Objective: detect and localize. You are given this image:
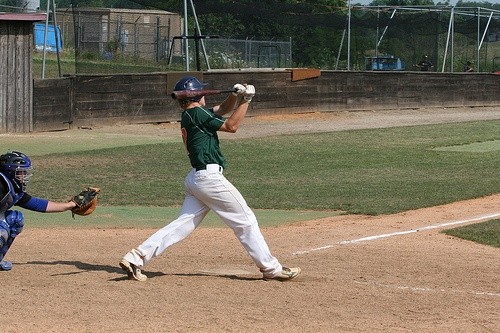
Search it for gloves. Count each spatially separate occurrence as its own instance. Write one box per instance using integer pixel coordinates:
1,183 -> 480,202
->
243,84 -> 255,101
232,83 -> 246,97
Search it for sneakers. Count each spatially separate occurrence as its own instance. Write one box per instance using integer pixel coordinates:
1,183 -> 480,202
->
119,258 -> 147,281
263,266 -> 301,281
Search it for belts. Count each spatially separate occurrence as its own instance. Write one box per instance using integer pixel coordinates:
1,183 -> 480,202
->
195,166 -> 221,172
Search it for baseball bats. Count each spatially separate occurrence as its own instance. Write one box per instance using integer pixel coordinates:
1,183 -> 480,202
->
170,87 -> 237,98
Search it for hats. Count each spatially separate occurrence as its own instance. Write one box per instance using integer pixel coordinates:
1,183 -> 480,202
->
0,153 -> 18,172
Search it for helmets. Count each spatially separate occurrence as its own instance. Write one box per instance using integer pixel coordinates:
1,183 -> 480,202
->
175,77 -> 208,105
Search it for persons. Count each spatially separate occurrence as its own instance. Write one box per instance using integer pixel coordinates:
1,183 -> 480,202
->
414,55 -> 434,71
464,61 -> 473,72
119,77 -> 301,282
0,151 -> 100,270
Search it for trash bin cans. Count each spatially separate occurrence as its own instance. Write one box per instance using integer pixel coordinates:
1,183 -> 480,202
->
33,24 -> 63,54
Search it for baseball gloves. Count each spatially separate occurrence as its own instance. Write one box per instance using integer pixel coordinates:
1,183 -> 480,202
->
69,186 -> 100,215
